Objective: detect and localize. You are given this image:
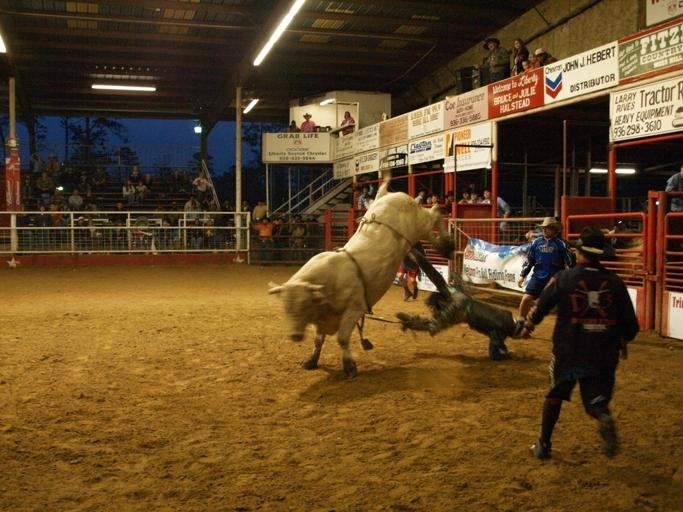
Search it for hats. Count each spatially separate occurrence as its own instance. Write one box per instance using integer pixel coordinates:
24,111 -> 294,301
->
538,217 -> 563,231
577,226 -> 606,254
482,38 -> 500,50
303,113 -> 312,118
534,47 -> 546,55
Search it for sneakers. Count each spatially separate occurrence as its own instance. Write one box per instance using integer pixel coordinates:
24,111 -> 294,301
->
531,438 -> 553,459
598,415 -> 620,454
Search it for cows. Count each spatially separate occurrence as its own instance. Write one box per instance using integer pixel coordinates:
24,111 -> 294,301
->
266,168 -> 457,379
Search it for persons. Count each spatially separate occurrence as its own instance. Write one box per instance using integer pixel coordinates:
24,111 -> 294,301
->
251,213 -> 306,261
514,217 -> 572,337
414,189 -> 511,232
354,174 -> 376,212
664,166 -> 683,213
395,249 -> 525,360
341,111 -> 355,136
473,33 -> 557,86
301,113 -> 317,133
13,153 -> 267,250
521,228 -> 640,460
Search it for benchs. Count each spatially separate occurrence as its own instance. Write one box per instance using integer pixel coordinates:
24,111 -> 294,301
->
20,175 -> 197,245
